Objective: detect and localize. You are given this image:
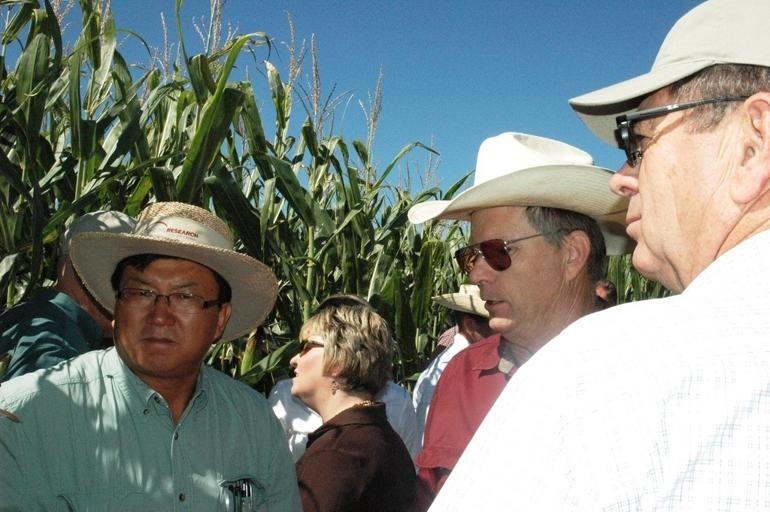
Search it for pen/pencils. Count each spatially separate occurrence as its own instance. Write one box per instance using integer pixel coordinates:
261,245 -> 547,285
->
228,479 -> 254,512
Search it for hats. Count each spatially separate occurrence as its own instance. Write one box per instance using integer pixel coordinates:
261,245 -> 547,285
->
569,0 -> 770,149
431,282 -> 490,317
408,130 -> 638,256
61,201 -> 278,344
318,293 -> 374,311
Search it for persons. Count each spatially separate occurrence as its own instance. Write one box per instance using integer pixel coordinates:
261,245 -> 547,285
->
0,195 -> 309,511
410,282 -> 499,448
264,290 -> 422,486
285,291 -> 422,511
420,1 -> 770,512
405,129 -> 640,511
1,208 -> 141,386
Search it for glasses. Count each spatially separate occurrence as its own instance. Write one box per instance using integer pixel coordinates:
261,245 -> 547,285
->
615,96 -> 749,166
455,228 -> 568,276
116,289 -> 221,313
297,341 -> 323,351
